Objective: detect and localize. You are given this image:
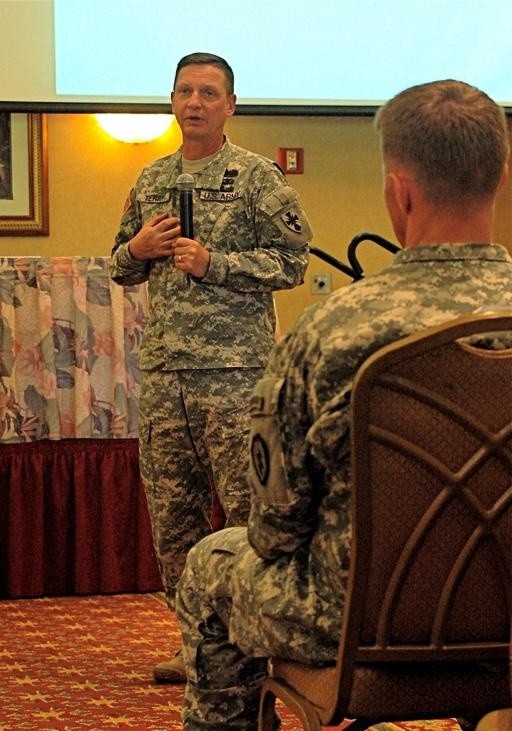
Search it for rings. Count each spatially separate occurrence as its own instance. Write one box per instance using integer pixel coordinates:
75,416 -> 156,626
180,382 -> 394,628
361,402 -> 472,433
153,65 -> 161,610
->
177,253 -> 181,263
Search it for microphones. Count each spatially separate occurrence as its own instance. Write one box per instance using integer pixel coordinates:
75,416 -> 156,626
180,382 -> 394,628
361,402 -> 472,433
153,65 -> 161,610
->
175,173 -> 197,240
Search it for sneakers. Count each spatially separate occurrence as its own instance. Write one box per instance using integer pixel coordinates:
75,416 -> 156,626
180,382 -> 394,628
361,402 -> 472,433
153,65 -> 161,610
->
154,650 -> 187,683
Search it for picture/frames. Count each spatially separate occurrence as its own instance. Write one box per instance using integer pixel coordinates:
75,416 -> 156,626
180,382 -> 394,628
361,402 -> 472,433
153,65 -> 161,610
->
1,112 -> 54,240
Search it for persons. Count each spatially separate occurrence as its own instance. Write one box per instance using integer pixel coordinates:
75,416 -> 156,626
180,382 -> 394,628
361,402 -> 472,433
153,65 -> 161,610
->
175,77 -> 512,730
107,50 -> 318,683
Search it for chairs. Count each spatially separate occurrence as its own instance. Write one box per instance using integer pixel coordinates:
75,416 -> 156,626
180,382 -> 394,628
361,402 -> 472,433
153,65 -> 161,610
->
252,312 -> 508,728
